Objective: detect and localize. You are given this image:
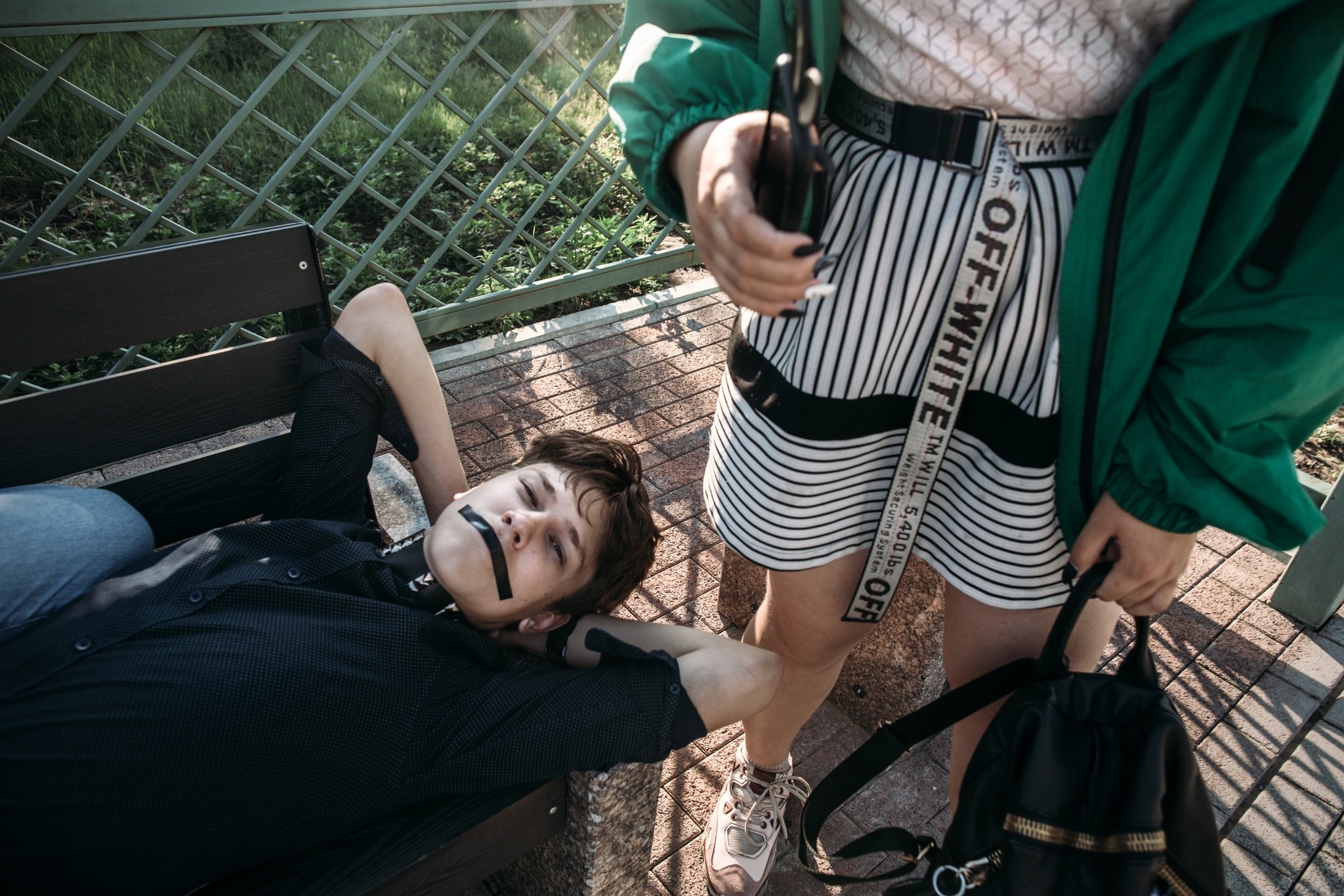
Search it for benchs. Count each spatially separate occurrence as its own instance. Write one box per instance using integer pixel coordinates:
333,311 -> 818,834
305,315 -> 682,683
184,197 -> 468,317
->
0,217 -> 382,546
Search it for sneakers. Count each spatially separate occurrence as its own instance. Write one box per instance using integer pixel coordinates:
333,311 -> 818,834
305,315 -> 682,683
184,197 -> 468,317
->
700,739 -> 812,896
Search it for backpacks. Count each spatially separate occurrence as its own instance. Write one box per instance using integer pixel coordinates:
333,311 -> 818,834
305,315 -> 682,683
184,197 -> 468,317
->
793,556 -> 1225,896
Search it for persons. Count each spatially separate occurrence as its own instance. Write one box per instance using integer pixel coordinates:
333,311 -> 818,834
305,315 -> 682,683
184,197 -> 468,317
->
0,282 -> 776,896
607,0 -> 1344,896
